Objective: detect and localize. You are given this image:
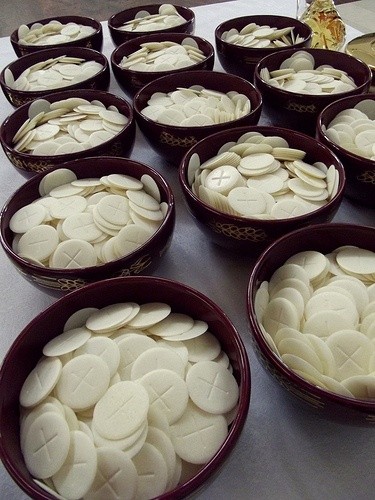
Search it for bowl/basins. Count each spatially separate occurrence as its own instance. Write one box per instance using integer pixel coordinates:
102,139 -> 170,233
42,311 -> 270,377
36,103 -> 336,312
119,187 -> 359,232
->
0,275 -> 250,500
178,124 -> 345,252
0,47 -> 111,110
132,69 -> 263,164
0,156 -> 175,303
245,222 -> 375,429
0,86 -> 139,183
314,92 -> 375,210
9,15 -> 104,58
106,4 -> 196,50
214,14 -> 312,88
254,47 -> 373,137
108,32 -> 215,100
